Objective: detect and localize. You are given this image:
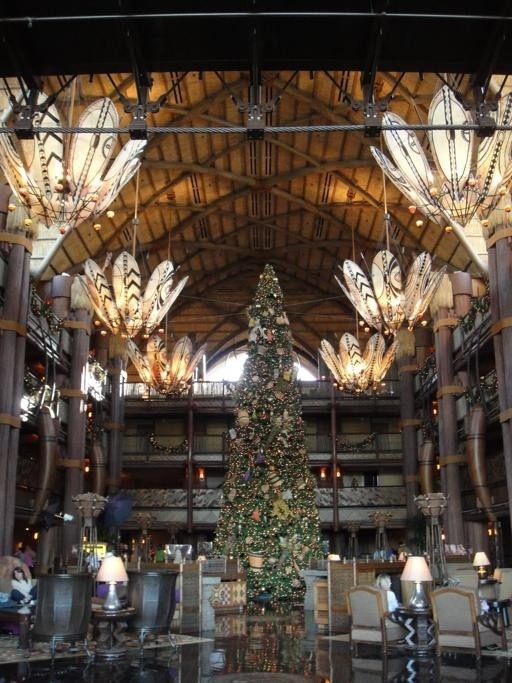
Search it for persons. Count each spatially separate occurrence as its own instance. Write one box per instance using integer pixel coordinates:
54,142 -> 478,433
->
11,567 -> 37,606
48,557 -> 67,573
19,542 -> 37,579
478,584 -> 499,615
376,573 -> 402,621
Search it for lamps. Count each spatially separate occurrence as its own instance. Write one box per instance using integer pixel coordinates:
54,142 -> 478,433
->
316,208 -> 401,395
76,167 -> 190,339
97,556 -> 130,611
0,75 -> 148,235
399,555 -> 434,612
126,207 -> 207,397
369,71 -> 512,233
472,551 -> 492,581
331,128 -> 447,335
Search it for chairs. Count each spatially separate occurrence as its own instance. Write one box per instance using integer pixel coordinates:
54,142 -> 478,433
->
299,561 -> 512,681
